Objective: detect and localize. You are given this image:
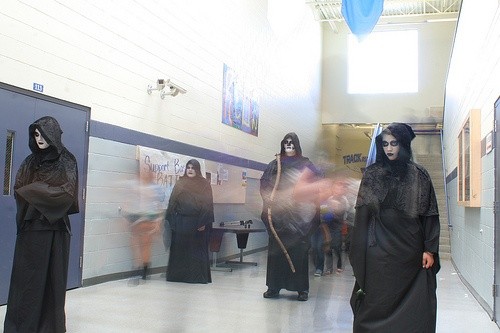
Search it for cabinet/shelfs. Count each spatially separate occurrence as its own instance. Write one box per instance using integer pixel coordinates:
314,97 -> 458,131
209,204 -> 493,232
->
456,108 -> 481,207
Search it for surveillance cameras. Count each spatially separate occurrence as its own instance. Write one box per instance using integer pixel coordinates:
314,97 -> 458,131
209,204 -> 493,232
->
157,79 -> 165,90
165,79 -> 187,94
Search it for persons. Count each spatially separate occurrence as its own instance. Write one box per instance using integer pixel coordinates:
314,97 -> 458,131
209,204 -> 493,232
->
164,159 -> 215,284
4,116 -> 81,333
349,122 -> 441,333
260,132 -> 319,301
311,183 -> 349,275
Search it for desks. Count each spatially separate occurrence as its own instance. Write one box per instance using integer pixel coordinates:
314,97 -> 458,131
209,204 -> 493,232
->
211,226 -> 266,272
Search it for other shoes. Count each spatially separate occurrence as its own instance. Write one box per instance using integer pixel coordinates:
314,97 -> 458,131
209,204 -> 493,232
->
337,269 -> 342,272
314,269 -> 323,276
325,270 -> 333,274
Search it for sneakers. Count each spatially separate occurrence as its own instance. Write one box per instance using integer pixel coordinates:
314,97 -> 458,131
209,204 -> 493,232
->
263,289 -> 279,298
298,291 -> 308,301
